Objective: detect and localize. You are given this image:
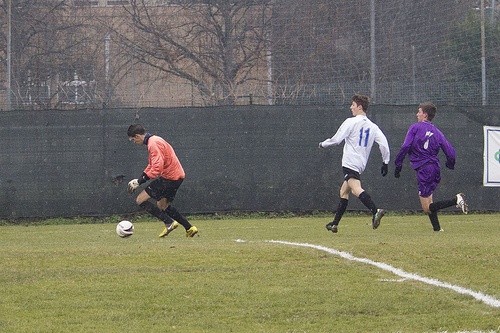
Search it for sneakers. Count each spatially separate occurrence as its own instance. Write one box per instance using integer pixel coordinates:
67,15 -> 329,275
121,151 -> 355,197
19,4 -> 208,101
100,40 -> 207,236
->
456,193 -> 469,214
372,208 -> 384,230
160,221 -> 179,238
186,226 -> 198,238
326,223 -> 337,233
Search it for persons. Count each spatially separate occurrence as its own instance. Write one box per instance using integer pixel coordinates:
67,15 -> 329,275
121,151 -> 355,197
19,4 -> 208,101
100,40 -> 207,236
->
319,95 -> 390,233
395,102 -> 468,231
127,124 -> 197,237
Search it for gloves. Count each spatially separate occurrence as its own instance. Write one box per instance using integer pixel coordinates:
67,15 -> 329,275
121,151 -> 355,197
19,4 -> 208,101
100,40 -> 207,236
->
394,167 -> 401,178
381,163 -> 388,176
127,179 -> 139,193
446,162 -> 456,169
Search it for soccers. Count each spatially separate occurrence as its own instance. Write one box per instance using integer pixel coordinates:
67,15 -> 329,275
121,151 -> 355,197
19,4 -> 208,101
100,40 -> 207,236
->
116,220 -> 134,238
